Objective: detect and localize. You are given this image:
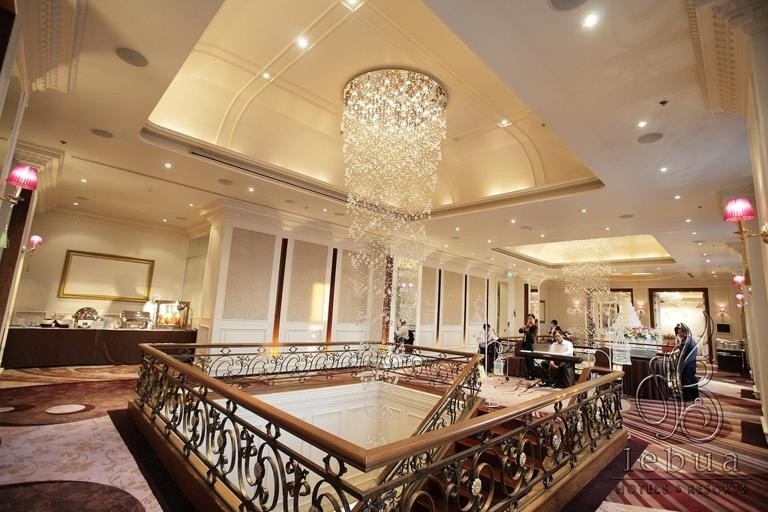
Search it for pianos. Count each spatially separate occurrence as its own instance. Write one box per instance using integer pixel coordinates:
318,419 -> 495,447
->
520,350 -> 584,363
657,349 -> 680,357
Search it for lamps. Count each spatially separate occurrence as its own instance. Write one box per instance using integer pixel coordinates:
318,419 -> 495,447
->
340,68 -> 450,331
718,197 -> 768,318
552,238 -> 613,348
0,163 -> 40,205
22,235 -> 43,253
177,304 -> 193,330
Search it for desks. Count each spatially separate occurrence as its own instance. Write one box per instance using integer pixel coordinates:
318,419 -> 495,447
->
0,327 -> 197,369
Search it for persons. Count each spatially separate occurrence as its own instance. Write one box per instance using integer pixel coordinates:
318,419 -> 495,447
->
548,320 -> 566,343
478,324 -> 499,373
674,324 -> 698,401
395,318 -> 413,345
519,313 -> 538,380
541,330 -> 574,384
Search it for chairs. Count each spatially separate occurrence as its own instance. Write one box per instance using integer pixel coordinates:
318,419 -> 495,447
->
593,347 -> 613,390
504,339 -> 528,377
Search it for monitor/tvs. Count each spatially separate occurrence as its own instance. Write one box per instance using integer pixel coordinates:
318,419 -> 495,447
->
717,323 -> 730,332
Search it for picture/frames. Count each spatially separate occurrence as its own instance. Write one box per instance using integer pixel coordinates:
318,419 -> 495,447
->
57,248 -> 155,303
